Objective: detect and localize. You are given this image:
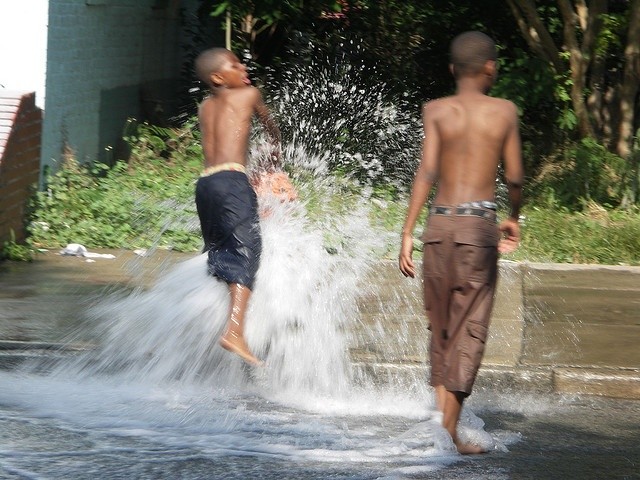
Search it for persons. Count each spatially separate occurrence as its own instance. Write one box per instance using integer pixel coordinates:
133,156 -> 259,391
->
192,47 -> 284,368
398,31 -> 524,456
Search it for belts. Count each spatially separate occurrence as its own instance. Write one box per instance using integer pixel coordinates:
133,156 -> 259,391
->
429,205 -> 497,221
